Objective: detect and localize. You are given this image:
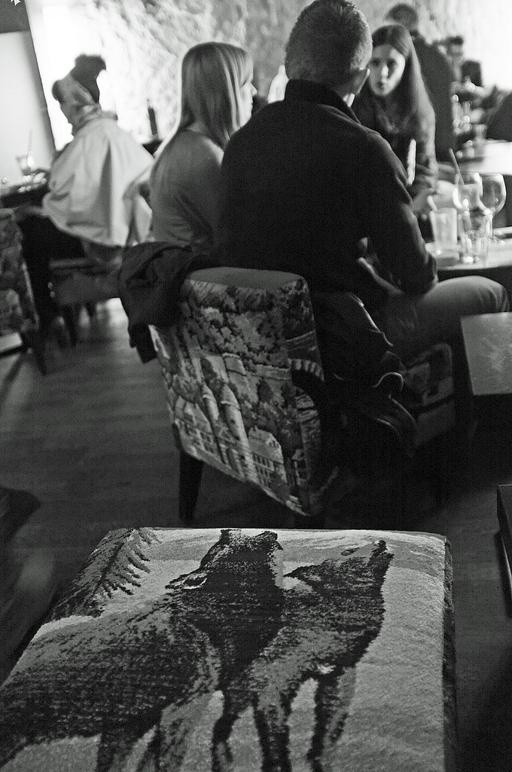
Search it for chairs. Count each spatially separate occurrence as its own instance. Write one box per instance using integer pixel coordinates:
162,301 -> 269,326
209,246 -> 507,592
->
144,247 -> 480,528
0,182 -> 165,377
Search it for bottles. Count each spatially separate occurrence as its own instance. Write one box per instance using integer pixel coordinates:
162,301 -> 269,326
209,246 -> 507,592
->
142,99 -> 165,139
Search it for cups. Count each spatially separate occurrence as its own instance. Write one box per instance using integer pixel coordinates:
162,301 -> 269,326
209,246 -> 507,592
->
15,150 -> 41,187
428,168 -> 508,265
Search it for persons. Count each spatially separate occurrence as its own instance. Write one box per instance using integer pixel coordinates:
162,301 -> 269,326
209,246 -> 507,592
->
15,52 -> 157,343
151,2 -> 512,488
